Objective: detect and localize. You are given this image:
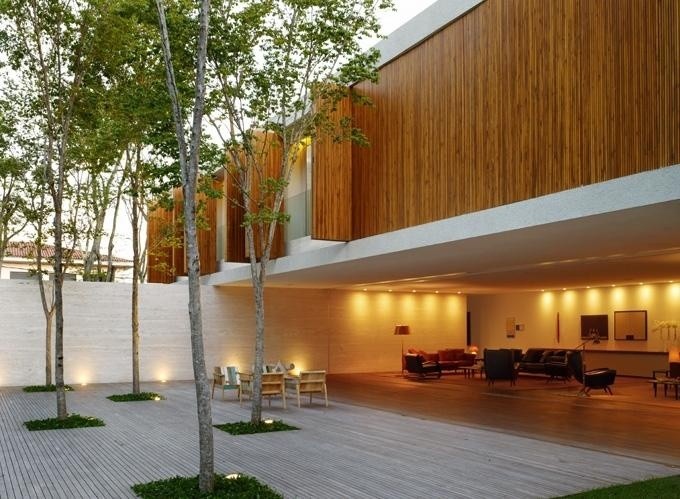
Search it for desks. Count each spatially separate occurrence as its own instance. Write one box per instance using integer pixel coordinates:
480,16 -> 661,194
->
238,371 -> 253,398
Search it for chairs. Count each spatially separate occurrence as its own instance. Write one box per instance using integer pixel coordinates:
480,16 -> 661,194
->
290,368 -> 330,410
256,373 -> 288,411
211,366 -> 241,402
253,363 -> 290,399
402,346 -> 680,403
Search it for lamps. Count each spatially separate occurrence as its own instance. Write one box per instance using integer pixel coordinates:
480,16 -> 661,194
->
393,325 -> 412,377
